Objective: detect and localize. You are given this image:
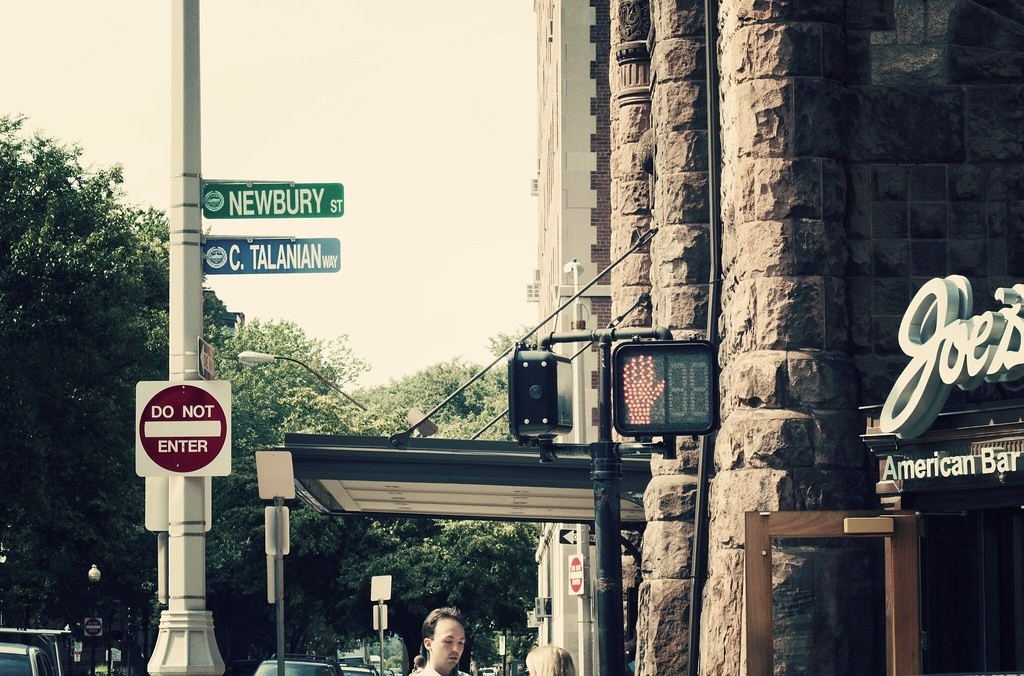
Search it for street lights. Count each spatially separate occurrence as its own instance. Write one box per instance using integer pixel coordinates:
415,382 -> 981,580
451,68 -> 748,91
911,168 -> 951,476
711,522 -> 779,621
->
88,565 -> 101,676
234,348 -> 372,415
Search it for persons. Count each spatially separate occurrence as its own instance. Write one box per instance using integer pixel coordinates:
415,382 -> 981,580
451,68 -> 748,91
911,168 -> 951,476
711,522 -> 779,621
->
408,607 -> 470,676
408,656 -> 427,676
526,646 -> 575,676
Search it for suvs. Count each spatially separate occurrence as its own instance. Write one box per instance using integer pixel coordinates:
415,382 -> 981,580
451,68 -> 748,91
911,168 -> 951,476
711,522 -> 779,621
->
0,642 -> 56,676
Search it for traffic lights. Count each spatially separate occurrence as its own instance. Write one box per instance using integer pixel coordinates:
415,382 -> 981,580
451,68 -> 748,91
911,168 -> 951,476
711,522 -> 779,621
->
613,340 -> 717,435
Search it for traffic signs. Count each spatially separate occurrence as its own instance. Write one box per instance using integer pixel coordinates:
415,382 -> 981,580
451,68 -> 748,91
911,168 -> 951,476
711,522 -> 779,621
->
199,235 -> 340,274
200,179 -> 346,218
195,337 -> 214,380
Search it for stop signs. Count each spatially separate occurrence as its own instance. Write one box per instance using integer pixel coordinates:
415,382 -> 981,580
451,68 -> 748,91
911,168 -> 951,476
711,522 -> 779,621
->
134,380 -> 233,476
84,617 -> 102,637
